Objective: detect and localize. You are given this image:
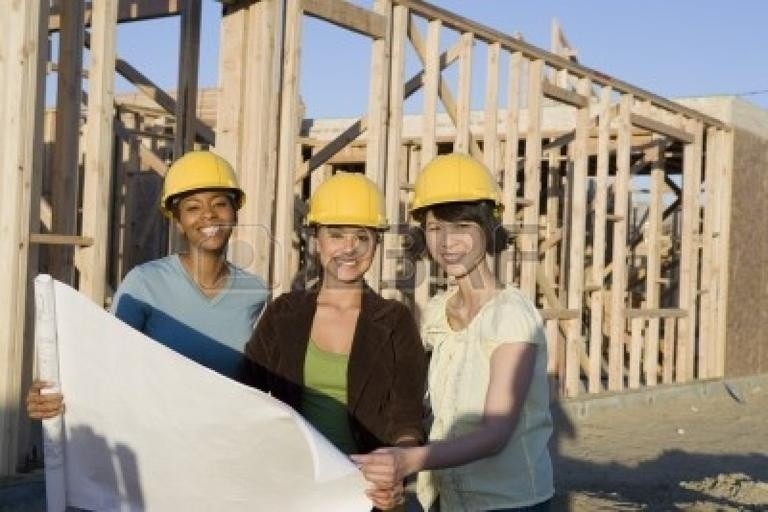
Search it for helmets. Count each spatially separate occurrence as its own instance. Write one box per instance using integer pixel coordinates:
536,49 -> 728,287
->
301,172 -> 388,232
158,150 -> 246,218
405,151 -> 504,220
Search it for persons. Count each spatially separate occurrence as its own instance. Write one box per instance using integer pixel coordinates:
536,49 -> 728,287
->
235,172 -> 432,511
348,151 -> 555,512
25,149 -> 271,419
